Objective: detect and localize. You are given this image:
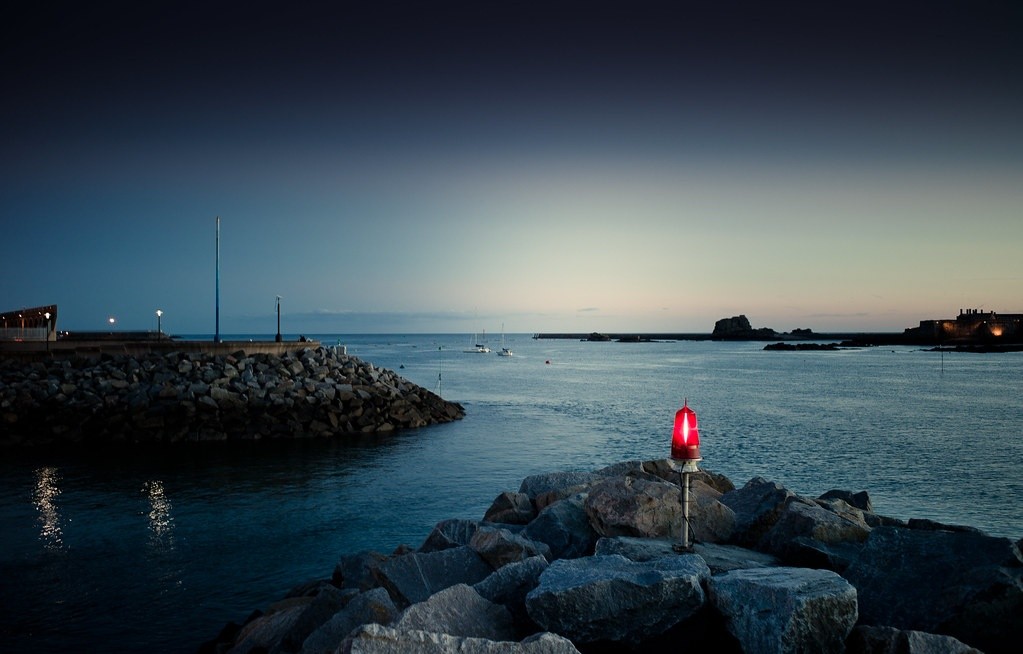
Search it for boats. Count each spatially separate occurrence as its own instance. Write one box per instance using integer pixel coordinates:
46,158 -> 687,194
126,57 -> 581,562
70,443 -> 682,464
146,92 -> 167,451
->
496,348 -> 513,357
462,345 -> 492,353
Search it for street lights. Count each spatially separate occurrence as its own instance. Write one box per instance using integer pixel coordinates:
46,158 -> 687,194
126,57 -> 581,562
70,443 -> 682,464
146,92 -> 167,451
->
44,312 -> 51,349
156,309 -> 163,342
